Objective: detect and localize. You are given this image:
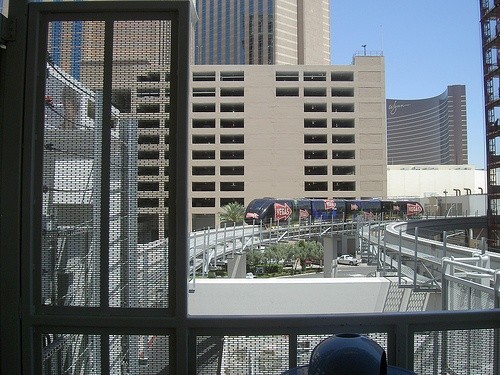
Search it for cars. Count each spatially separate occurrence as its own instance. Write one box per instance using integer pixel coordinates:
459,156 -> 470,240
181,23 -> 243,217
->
337,254 -> 362,266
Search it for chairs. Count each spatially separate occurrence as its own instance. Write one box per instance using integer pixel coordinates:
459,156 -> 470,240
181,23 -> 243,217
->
310,333 -> 387,365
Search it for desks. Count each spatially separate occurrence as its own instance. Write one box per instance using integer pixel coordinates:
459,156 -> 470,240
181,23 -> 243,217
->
281,365 -> 415,375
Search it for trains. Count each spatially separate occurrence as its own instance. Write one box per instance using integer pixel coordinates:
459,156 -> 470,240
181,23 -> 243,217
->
245,197 -> 423,225
